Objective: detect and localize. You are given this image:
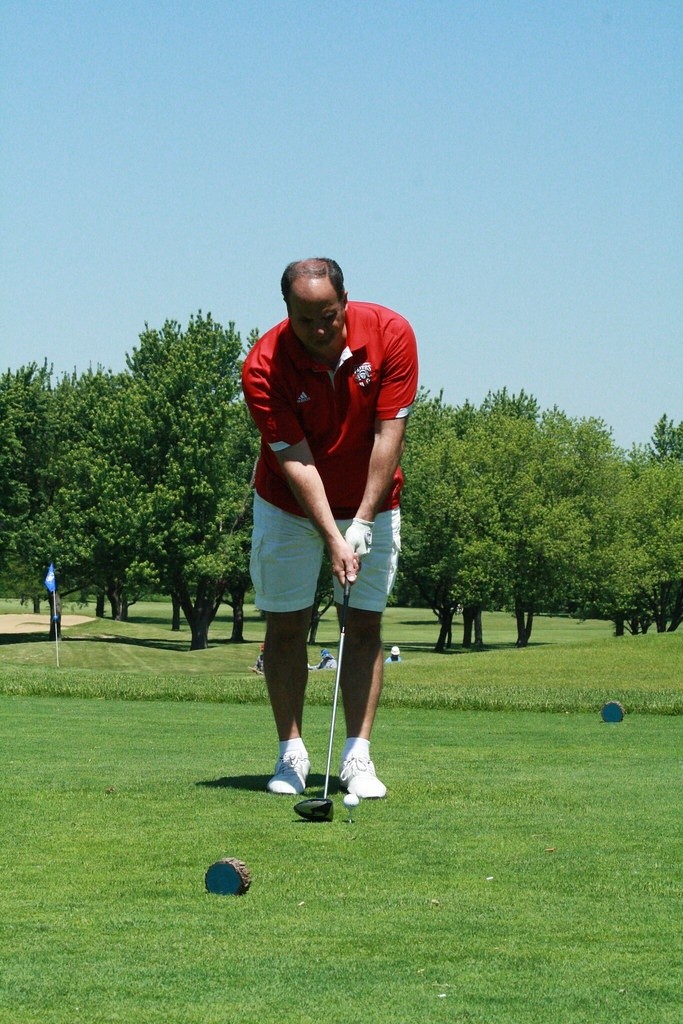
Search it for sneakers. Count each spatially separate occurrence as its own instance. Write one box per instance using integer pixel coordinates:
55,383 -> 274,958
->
266,752 -> 313,796
337,767 -> 388,800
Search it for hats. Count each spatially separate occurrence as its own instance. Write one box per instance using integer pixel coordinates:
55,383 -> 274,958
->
321,649 -> 334,659
390,646 -> 400,656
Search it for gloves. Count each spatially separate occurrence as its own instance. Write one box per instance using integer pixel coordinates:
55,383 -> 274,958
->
344,518 -> 375,562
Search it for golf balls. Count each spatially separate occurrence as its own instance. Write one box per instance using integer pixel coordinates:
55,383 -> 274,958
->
343,794 -> 359,808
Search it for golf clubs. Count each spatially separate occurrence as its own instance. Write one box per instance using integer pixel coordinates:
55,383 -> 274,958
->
292,574 -> 351,823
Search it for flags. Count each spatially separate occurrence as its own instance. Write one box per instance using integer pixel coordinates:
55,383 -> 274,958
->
44,562 -> 57,593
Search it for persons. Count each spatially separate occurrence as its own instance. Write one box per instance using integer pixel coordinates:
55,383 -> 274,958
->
308,648 -> 338,672
239,254 -> 422,802
383,644 -> 401,664
251,641 -> 268,676
